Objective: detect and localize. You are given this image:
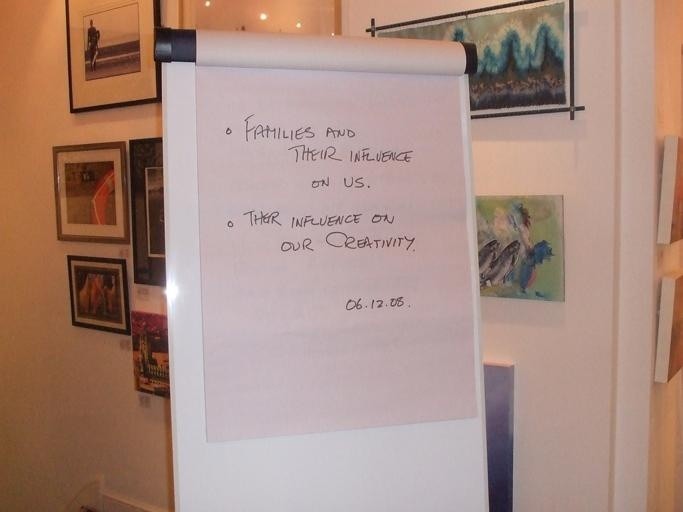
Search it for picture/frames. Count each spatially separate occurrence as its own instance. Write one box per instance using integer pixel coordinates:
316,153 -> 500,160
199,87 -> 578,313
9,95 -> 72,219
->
482,362 -> 513,511
64,3 -> 161,112
129,138 -> 165,285
471,192 -> 567,304
653,130 -> 683,245
66,255 -> 130,336
54,141 -> 129,242
653,274 -> 682,386
360,0 -> 589,128
